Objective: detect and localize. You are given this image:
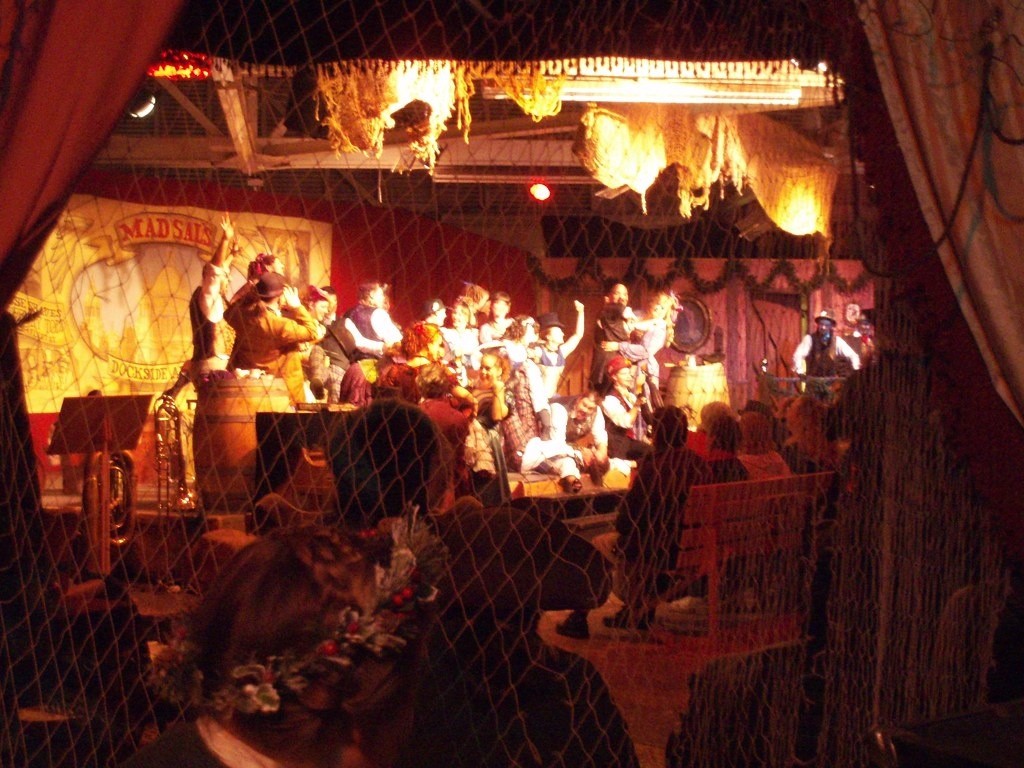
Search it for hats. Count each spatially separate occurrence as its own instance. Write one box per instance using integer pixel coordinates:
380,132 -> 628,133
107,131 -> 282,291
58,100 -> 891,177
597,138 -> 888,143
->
538,313 -> 565,329
815,309 -> 836,327
420,298 -> 445,318
607,355 -> 631,376
256,272 -> 287,298
738,400 -> 769,420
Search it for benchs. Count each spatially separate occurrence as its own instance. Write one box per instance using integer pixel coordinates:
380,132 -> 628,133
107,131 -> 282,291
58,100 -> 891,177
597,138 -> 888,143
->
616,470 -> 835,644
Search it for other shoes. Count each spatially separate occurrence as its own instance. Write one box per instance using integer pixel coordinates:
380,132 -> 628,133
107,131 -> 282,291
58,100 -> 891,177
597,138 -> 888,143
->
556,611 -> 590,639
671,594 -> 708,615
309,377 -> 326,400
603,607 -> 654,630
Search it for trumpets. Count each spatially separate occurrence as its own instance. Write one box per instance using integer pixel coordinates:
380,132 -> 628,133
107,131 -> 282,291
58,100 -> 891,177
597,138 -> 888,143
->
151,394 -> 197,512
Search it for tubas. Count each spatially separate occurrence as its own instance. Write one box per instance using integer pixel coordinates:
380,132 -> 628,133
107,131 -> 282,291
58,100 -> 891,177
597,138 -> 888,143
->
85,448 -> 140,577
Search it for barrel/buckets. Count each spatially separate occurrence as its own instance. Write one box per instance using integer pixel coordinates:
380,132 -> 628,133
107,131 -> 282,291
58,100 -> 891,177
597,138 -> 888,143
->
666,364 -> 730,432
194,376 -> 293,514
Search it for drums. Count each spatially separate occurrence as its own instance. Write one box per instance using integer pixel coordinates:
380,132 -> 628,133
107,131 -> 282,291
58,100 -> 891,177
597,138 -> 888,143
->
664,362 -> 731,428
192,377 -> 277,512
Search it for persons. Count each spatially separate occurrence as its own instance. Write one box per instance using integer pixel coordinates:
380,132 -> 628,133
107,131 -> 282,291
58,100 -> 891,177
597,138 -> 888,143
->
790,311 -> 862,378
0,397 -> 643,768
837,302 -> 879,367
163,211 -> 675,490
605,367 -> 879,644
